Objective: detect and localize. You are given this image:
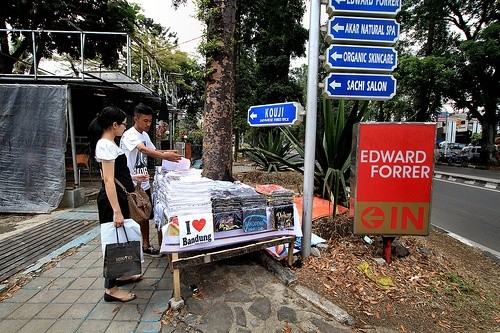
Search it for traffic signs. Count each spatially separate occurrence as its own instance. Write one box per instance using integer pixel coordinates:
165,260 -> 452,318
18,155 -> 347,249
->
246,98 -> 306,129
324,0 -> 397,105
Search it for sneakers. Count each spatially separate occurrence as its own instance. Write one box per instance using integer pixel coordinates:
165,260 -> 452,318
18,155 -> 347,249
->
142,246 -> 162,257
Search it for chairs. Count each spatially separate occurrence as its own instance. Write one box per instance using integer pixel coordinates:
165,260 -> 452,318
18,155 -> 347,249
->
66,152 -> 93,187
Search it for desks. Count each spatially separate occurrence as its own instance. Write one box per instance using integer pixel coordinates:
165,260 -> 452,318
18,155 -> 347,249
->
160,228 -> 296,300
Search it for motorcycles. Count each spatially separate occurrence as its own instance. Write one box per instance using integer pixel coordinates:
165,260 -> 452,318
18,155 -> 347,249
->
437,150 -> 469,166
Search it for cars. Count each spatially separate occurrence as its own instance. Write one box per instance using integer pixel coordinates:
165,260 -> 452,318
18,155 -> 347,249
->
435,140 -> 481,158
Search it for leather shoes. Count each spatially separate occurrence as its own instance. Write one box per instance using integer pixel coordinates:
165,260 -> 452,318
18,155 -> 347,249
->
104,291 -> 136,302
115,274 -> 143,287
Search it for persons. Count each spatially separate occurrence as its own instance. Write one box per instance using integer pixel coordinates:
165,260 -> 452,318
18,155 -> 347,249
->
119,103 -> 185,257
88,106 -> 150,303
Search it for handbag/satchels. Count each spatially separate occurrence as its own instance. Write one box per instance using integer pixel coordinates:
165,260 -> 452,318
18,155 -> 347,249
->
126,185 -> 153,222
100,219 -> 144,267
103,224 -> 142,279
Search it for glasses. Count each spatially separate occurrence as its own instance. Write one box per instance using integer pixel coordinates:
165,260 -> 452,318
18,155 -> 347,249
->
121,122 -> 127,127
